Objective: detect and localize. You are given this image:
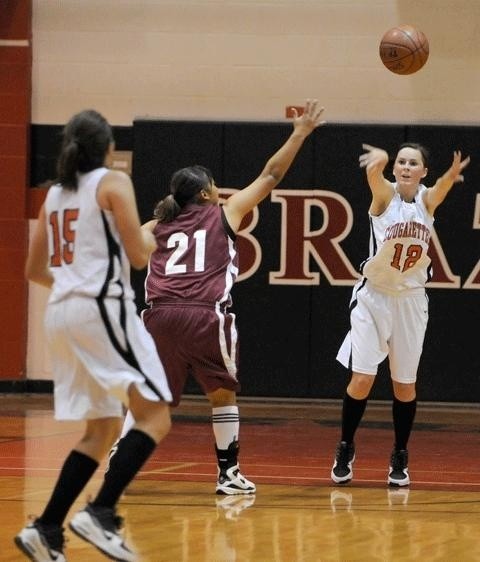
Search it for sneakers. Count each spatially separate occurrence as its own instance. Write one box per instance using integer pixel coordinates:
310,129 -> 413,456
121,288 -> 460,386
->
67,495 -> 137,562
330,441 -> 356,484
387,448 -> 410,487
215,462 -> 256,496
13,514 -> 66,562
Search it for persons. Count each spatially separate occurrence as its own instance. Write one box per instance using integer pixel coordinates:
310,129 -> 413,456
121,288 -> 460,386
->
14,111 -> 173,562
331,142 -> 470,487
103,98 -> 328,495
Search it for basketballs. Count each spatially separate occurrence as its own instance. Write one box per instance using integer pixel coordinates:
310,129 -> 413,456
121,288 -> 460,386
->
380,24 -> 428,74
